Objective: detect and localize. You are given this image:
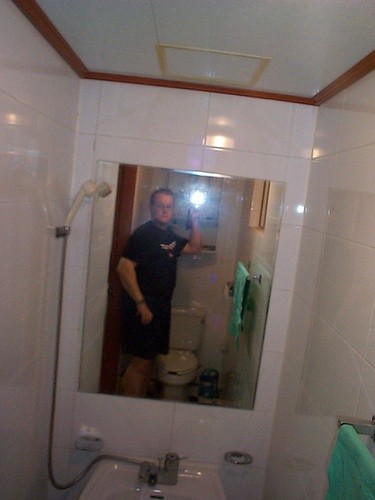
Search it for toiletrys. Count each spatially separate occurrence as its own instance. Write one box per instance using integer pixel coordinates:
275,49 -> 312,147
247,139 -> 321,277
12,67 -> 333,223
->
224,281 -> 234,300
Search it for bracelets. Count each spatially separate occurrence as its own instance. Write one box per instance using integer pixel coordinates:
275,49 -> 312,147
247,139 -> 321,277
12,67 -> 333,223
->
135,299 -> 145,305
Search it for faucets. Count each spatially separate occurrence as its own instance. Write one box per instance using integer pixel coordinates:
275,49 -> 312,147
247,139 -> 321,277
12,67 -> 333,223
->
137,458 -> 159,486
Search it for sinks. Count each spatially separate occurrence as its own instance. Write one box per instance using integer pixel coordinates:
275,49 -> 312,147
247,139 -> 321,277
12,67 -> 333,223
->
77,471 -> 223,499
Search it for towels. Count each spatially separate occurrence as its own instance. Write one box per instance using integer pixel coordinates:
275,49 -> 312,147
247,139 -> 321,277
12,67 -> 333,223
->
323,424 -> 375,500
228,261 -> 250,353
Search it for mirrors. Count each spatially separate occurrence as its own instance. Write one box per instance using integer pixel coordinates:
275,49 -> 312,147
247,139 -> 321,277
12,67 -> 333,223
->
76,154 -> 287,412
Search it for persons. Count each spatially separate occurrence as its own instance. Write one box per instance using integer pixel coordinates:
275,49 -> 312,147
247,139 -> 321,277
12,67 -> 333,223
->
115,188 -> 202,398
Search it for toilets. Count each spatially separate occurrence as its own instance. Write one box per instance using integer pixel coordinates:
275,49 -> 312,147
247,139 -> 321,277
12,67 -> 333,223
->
154,308 -> 205,401
